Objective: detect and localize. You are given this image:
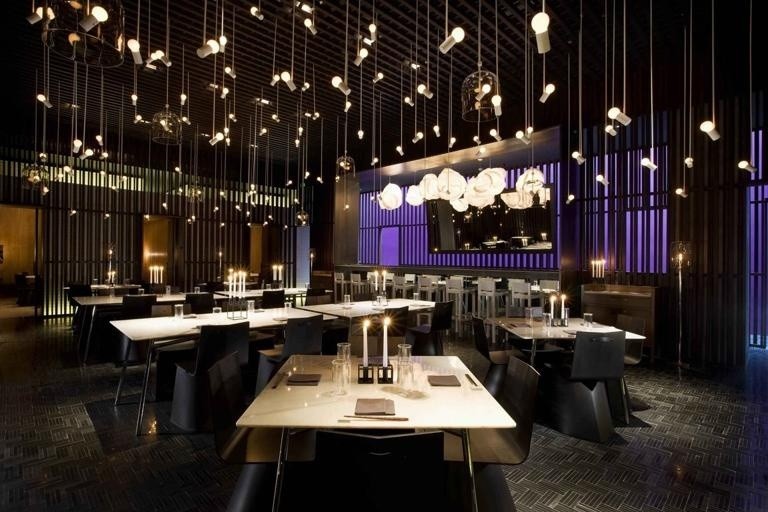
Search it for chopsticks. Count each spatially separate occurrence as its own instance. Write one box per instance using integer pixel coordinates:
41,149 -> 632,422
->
465,374 -> 478,386
344,415 -> 408,421
272,371 -> 285,389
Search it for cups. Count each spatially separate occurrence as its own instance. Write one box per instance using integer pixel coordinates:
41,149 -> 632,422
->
246,300 -> 255,316
343,294 -> 351,308
138,289 -> 144,295
174,304 -> 183,321
194,286 -> 200,294
331,359 -> 351,395
113,278 -> 129,287
583,312 -> 592,327
336,342 -> 351,361
93,278 -> 108,286
412,292 -> 419,306
541,313 -> 552,334
397,360 -> 414,388
165,285 -> 171,296
376,296 -> 383,309
213,307 -> 222,314
397,343 -> 411,365
284,302 -> 291,315
109,287 -> 115,299
